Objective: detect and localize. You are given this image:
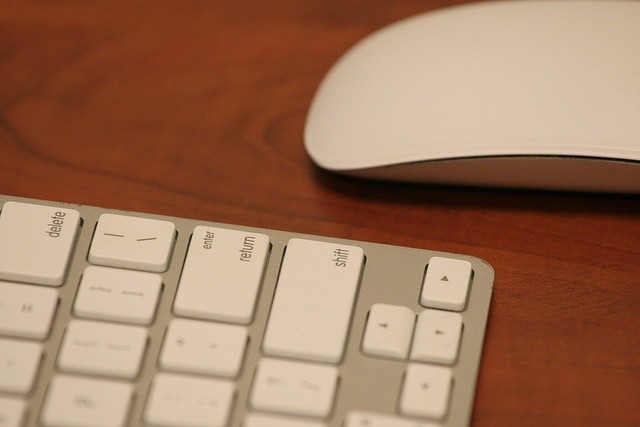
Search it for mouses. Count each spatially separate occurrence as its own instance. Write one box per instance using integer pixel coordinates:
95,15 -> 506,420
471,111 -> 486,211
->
304,0 -> 640,193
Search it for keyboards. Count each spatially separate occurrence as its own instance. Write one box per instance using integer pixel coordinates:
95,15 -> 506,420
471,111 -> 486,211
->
1,195 -> 496,427
